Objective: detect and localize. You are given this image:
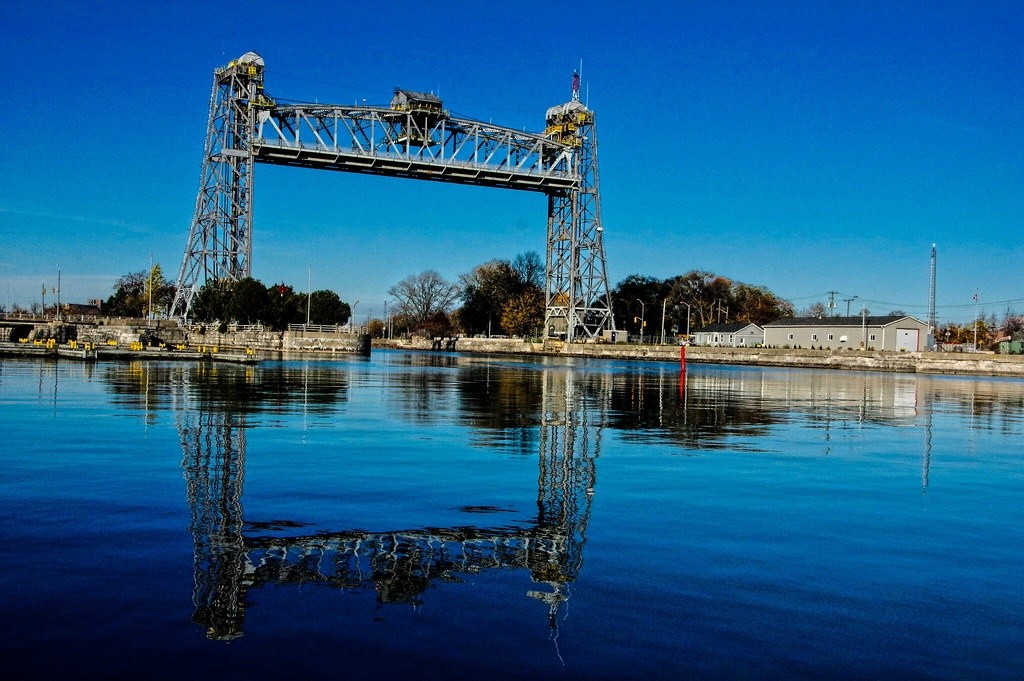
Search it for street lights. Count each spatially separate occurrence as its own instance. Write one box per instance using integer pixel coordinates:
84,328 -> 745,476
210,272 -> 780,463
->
307,263 -> 311,326
56,264 -> 61,321
660,298 -> 670,345
637,299 -> 644,345
680,301 -> 691,347
352,300 -> 360,333
